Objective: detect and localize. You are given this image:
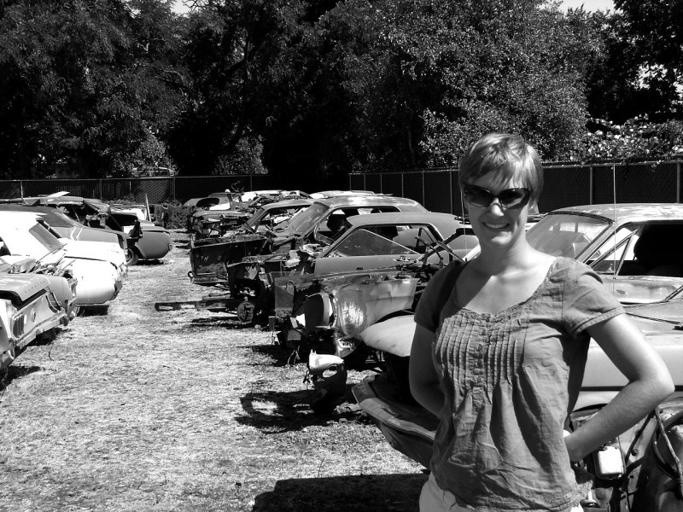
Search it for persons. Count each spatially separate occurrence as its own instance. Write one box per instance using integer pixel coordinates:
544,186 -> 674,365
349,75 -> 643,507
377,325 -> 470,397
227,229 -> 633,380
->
406,132 -> 676,512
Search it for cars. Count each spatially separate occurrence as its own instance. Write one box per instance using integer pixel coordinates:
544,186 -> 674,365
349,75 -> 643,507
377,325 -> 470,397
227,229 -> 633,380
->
0,191 -> 172,376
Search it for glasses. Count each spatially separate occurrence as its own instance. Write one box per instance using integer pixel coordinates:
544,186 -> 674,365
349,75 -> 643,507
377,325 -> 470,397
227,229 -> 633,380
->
462,180 -> 532,208
